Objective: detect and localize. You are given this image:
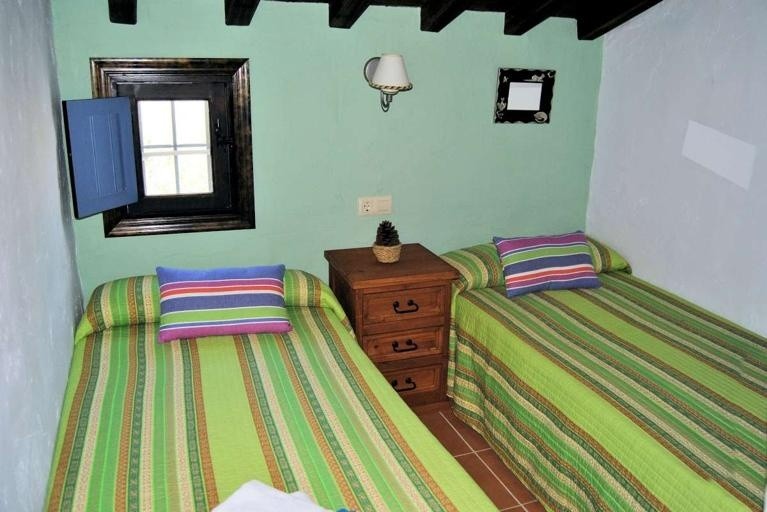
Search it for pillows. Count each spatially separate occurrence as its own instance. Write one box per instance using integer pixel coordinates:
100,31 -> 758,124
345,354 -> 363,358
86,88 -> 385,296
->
493,227 -> 601,299
154,263 -> 292,343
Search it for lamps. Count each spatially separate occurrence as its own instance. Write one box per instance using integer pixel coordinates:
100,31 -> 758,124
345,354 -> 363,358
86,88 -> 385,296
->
364,50 -> 413,110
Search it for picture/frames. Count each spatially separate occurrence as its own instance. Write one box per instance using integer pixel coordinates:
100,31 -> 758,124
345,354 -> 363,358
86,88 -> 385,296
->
492,67 -> 556,127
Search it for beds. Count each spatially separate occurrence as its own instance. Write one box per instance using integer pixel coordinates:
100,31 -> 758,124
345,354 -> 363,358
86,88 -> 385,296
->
438,229 -> 767,510
39,263 -> 497,510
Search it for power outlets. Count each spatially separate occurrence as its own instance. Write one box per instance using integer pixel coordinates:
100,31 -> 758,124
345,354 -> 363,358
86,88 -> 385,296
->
356,196 -> 373,216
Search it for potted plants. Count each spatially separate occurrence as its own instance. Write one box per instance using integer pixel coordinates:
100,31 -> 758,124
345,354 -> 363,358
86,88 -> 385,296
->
371,219 -> 400,263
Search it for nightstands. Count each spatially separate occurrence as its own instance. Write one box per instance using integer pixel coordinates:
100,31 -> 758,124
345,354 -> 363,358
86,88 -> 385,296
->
324,241 -> 460,404
